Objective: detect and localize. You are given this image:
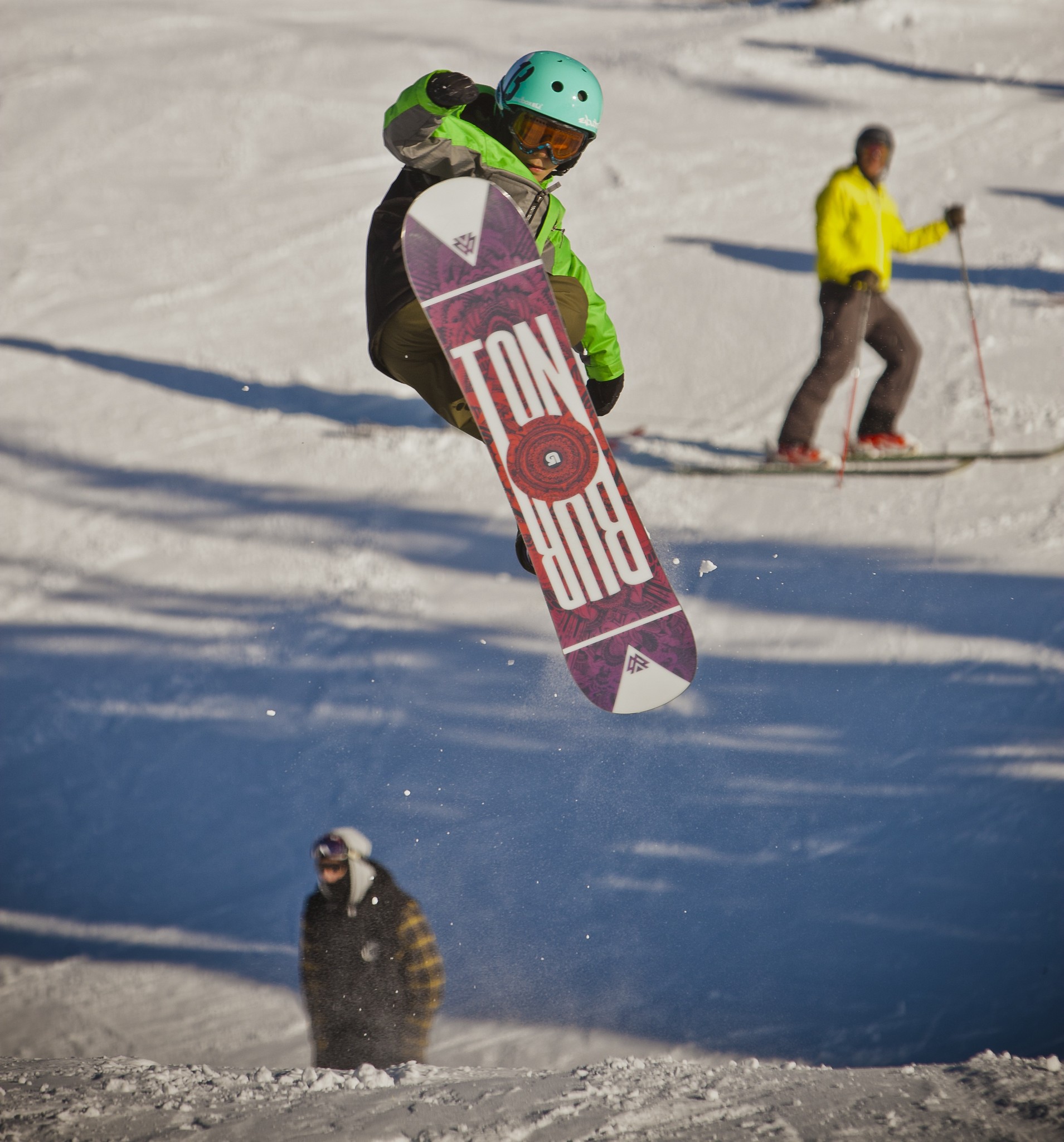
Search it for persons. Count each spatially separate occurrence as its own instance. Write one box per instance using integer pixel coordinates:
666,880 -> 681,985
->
780,127 -> 966,464
298,827 -> 442,1071
365,51 -> 623,572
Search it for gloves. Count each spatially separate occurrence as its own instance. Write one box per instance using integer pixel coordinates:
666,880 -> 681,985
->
586,373 -> 625,416
945,206 -> 965,229
426,72 -> 479,108
849,270 -> 878,291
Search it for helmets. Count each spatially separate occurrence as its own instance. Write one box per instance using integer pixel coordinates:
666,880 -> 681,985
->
494,50 -> 603,172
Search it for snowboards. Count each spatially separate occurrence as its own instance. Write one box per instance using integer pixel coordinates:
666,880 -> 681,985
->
400,176 -> 697,715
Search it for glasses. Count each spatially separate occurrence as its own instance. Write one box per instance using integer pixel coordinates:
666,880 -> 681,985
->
317,864 -> 341,872
507,107 -> 589,165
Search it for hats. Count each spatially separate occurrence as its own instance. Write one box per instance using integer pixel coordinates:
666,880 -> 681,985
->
309,826 -> 376,918
857,128 -> 890,147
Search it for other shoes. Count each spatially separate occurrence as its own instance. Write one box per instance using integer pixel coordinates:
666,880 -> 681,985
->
852,432 -> 925,458
778,444 -> 842,467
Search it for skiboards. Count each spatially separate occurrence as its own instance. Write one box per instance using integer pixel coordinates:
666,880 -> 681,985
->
670,442 -> 1063,477
